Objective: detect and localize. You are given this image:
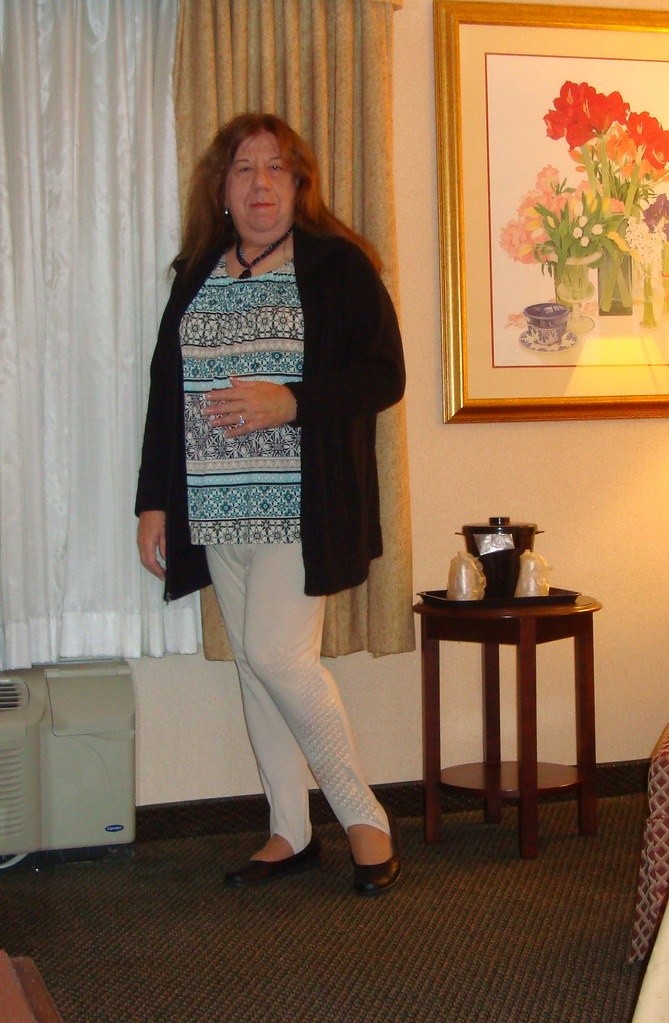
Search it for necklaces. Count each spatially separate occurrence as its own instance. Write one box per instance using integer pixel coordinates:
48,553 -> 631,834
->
235,224 -> 294,279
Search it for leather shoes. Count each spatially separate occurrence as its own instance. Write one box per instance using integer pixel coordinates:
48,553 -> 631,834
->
349,805 -> 402,895
224,837 -> 320,887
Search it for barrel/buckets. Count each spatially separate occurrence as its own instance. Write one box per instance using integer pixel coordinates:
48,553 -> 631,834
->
455,516 -> 544,596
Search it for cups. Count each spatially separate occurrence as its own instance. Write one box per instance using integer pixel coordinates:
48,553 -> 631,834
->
516,555 -> 548,595
449,557 -> 477,601
523,304 -> 569,344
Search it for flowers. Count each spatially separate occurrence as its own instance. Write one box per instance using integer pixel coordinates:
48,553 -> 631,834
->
626,193 -> 669,272
500,167 -> 641,306
543,78 -> 669,246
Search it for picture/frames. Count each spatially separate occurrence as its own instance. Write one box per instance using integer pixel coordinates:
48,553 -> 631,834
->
432,0 -> 668,424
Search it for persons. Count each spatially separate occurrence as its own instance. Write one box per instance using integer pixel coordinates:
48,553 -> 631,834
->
134,113 -> 408,894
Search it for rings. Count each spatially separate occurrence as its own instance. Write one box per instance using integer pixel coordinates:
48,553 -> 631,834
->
239,414 -> 245,423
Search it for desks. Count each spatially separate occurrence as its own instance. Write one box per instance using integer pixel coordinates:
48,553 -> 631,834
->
409,598 -> 604,861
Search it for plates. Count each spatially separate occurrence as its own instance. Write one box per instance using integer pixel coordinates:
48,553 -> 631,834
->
520,330 -> 577,353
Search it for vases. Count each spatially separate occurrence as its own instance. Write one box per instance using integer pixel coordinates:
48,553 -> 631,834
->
552,257 -> 600,333
595,215 -> 634,316
640,265 -> 668,330
